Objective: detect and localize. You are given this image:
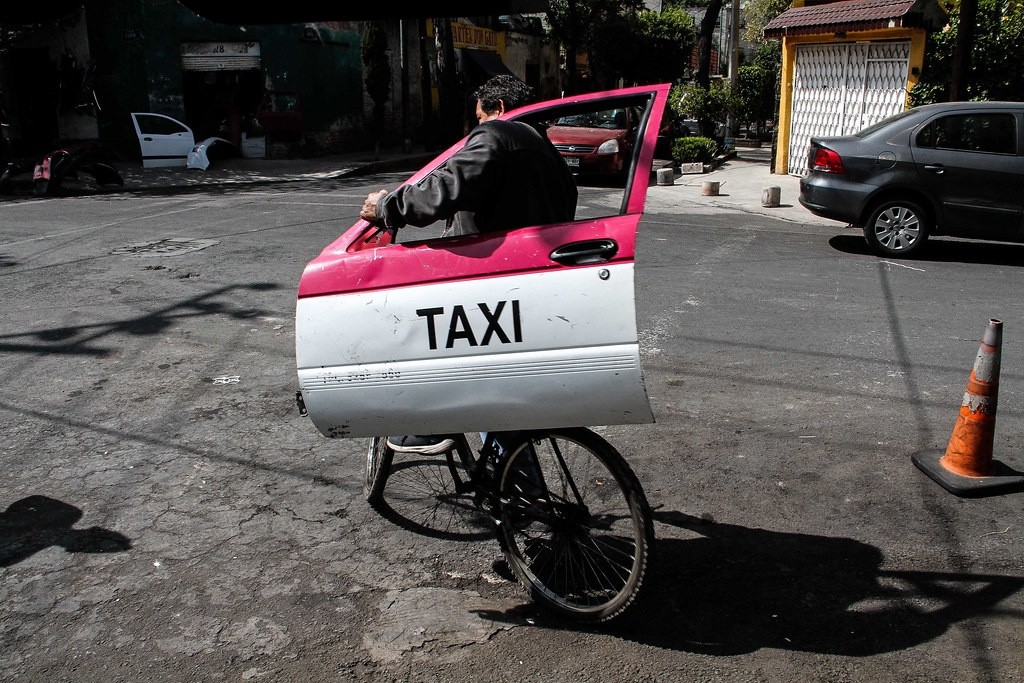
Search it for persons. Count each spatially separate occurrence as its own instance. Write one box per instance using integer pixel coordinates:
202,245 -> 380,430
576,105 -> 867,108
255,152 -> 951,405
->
361,73 -> 580,512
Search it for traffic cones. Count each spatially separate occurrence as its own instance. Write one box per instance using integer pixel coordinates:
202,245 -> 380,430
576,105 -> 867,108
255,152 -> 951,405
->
910,318 -> 1024,498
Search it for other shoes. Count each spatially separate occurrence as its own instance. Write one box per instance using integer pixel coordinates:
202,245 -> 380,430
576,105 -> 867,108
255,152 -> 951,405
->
386,435 -> 456,455
468,499 -> 548,529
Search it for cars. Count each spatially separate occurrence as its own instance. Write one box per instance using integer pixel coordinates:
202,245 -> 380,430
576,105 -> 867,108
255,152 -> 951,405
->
798,100 -> 1024,259
639,102 -> 690,151
542,105 -> 646,181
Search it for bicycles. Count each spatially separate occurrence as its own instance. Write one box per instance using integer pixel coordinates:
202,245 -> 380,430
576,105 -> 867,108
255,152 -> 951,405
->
363,423 -> 658,627
